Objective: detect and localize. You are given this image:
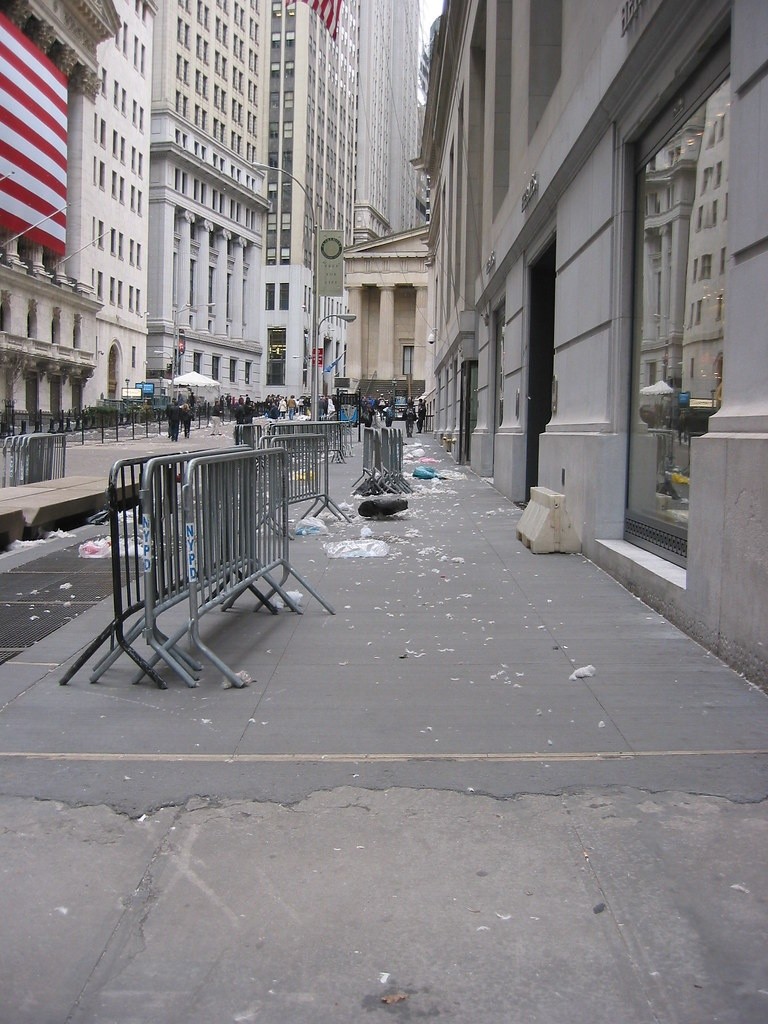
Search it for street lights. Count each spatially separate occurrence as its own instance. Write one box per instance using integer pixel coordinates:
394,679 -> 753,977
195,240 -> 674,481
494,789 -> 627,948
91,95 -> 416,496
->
244,164 -> 357,422
125,378 -> 130,407
154,302 -> 215,403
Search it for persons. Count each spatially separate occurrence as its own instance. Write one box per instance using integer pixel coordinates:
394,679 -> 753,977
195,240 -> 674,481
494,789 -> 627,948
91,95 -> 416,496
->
179,403 -> 195,438
402,401 -> 418,438
383,404 -> 394,427
416,398 -> 426,432
178,392 -> 387,430
167,398 -> 178,438
169,401 -> 179,441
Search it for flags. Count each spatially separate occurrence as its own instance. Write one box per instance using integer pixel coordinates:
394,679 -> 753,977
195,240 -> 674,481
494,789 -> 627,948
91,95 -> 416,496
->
0,13 -> 69,255
285,0 -> 343,41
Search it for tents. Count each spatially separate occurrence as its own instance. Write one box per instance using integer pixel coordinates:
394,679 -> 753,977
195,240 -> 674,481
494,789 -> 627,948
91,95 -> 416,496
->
174,371 -> 221,397
639,380 -> 677,429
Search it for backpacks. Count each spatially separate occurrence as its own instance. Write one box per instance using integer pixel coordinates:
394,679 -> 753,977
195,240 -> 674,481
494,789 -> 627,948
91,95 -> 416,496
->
406,408 -> 415,420
183,410 -> 191,421
